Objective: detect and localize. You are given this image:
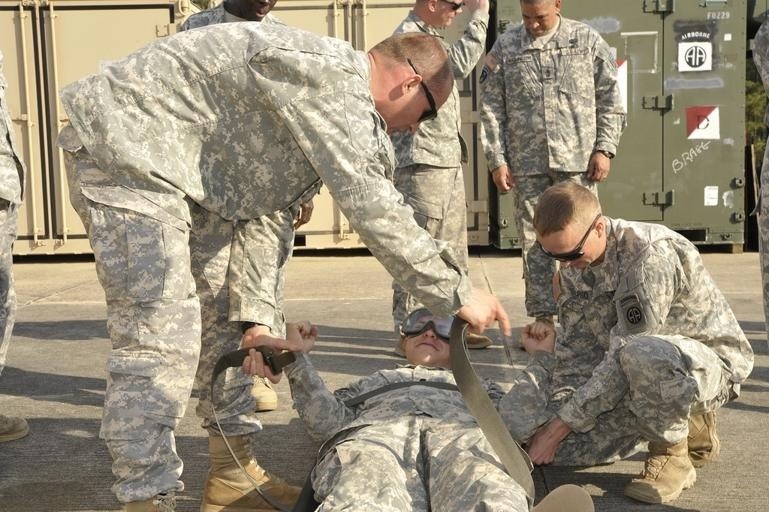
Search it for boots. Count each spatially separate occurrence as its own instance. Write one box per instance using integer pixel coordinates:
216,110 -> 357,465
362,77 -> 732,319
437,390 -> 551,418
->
201,435 -> 302,512
124,494 -> 174,510
626,438 -> 696,503
688,413 -> 719,470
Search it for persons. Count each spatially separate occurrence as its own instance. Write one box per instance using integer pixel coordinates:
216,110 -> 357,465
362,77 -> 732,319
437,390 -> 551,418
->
178,1 -> 315,415
524,180 -> 756,504
386,0 -> 495,358
54,18 -> 513,512
479,0 -> 626,351
283,305 -> 558,512
751,15 -> 769,341
0,48 -> 31,445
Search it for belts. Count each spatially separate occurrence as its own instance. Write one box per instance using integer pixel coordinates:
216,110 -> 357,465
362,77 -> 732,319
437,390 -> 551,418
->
0,199 -> 14,210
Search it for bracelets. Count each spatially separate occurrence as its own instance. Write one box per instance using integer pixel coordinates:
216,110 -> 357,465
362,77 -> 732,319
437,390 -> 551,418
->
239,320 -> 265,334
598,150 -> 614,159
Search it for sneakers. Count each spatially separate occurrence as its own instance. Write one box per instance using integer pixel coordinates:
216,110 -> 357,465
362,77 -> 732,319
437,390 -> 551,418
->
395,334 -> 409,359
465,332 -> 491,350
251,375 -> 279,411
529,485 -> 594,512
0,416 -> 30,441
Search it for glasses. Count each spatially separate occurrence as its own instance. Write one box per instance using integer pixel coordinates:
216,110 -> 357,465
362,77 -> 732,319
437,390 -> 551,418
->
538,211 -> 602,262
406,59 -> 438,122
440,0 -> 461,11
401,308 -> 454,343
